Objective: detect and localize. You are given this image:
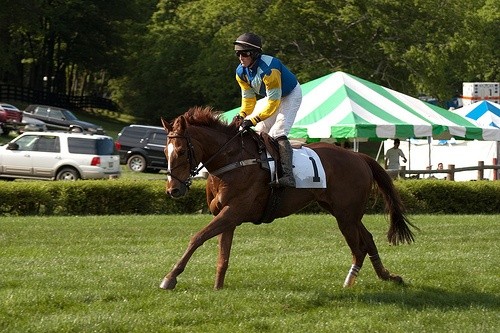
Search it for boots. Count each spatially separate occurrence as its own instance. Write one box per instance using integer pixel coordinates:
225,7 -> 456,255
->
269,136 -> 296,187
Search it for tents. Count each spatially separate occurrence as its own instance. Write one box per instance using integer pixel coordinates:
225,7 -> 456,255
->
216,72 -> 500,179
384,100 -> 500,182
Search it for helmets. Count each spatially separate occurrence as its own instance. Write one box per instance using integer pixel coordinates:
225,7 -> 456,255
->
233,33 -> 262,52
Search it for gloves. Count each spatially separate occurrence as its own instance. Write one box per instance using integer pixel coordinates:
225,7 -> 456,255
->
233,115 -> 244,126
241,119 -> 253,129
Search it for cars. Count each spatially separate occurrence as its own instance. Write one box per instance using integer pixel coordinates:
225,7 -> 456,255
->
0,103 -> 47,137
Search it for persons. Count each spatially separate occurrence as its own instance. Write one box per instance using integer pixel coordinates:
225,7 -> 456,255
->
230,32 -> 302,188
432,163 -> 448,180
385,139 -> 407,169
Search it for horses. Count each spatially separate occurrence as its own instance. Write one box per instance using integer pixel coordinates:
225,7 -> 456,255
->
159,105 -> 420,291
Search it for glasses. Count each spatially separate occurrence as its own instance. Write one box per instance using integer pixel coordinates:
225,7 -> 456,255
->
237,51 -> 254,57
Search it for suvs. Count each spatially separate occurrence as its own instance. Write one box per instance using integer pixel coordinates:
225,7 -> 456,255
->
21,104 -> 106,135
0,131 -> 123,181
115,124 -> 169,172
